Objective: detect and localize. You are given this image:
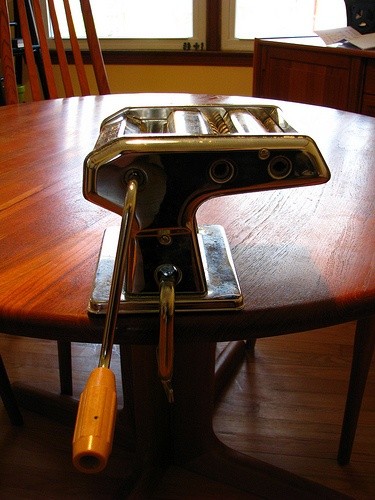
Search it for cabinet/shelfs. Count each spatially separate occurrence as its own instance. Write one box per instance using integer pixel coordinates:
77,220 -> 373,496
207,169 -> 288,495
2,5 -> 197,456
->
250,34 -> 374,117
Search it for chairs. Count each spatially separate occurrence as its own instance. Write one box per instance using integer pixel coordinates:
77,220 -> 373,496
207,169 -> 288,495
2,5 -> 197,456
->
0,1 -> 111,108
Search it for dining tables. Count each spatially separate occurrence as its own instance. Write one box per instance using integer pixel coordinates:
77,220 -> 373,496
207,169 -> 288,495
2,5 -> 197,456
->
1,89 -> 374,467
